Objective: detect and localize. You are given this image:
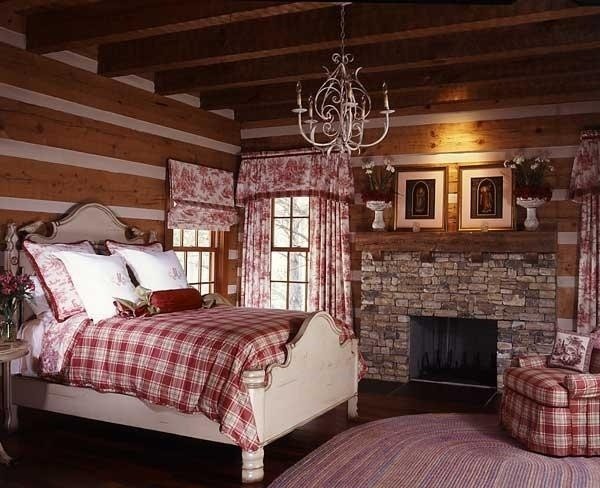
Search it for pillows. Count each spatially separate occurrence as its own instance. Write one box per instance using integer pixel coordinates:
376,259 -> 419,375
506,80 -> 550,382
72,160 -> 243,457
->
18,237 -> 203,324
548,328 -> 597,373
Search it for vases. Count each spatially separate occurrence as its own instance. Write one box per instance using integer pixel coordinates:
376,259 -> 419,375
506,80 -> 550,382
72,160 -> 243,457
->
516,197 -> 549,231
0,319 -> 17,344
365,201 -> 391,230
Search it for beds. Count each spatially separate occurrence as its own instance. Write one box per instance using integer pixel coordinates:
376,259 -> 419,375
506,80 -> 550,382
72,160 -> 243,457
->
5,202 -> 360,483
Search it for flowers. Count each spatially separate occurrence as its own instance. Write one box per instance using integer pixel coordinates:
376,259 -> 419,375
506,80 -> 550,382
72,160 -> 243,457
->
359,159 -> 396,202
504,150 -> 554,201
0,272 -> 37,342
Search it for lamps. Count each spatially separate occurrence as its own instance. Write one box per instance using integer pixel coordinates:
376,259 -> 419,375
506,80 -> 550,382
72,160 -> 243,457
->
290,2 -> 396,159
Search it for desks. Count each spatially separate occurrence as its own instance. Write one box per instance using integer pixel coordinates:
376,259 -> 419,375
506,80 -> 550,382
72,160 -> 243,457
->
0,340 -> 30,467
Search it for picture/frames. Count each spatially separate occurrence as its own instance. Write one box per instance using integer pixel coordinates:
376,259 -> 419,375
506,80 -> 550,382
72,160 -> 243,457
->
456,164 -> 518,232
394,167 -> 448,232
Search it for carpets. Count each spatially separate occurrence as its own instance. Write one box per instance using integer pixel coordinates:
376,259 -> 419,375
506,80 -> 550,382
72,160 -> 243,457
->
266,413 -> 600,488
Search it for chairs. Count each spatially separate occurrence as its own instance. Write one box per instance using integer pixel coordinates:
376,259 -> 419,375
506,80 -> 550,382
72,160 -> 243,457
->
498,349 -> 600,457
